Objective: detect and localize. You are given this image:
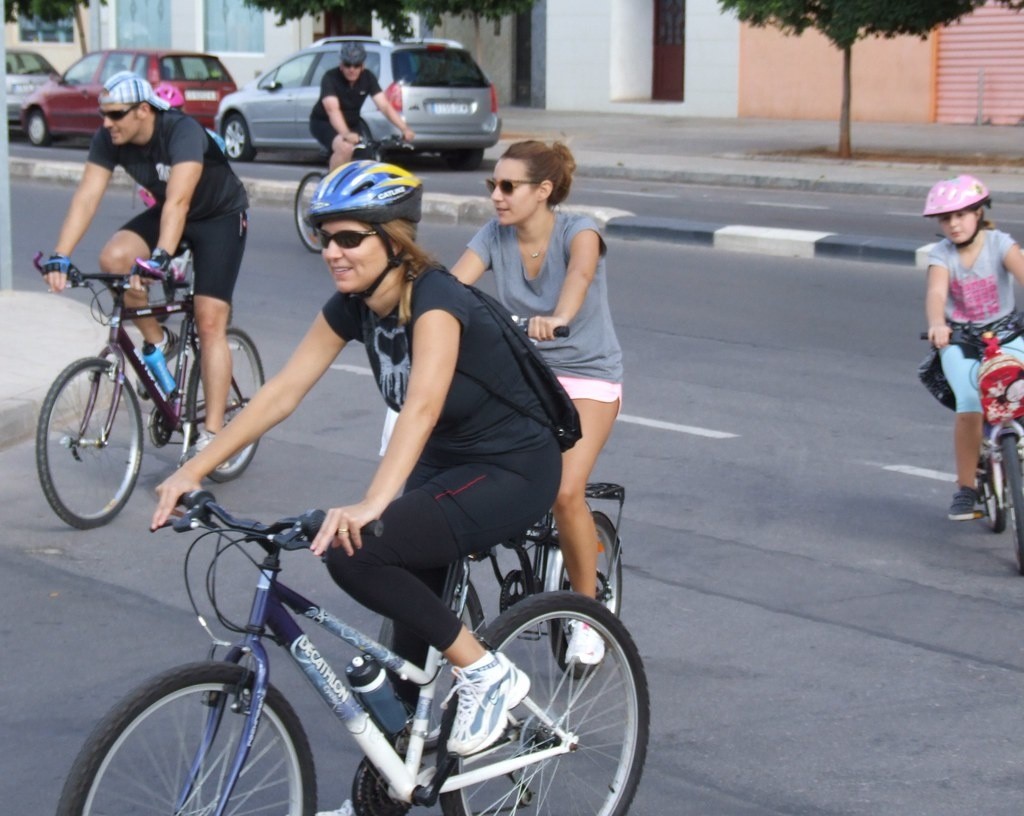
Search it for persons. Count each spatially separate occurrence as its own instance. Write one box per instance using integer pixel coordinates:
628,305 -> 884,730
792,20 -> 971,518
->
148,156 -> 561,816
309,41 -> 414,173
40,71 -> 247,469
449,140 -> 622,665
913,173 -> 1024,519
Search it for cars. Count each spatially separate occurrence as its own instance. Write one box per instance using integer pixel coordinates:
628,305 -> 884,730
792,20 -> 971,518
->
213,36 -> 503,166
21,47 -> 235,158
7,46 -> 65,142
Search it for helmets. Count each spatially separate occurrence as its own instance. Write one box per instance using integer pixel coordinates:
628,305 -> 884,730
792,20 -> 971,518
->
303,160 -> 423,227
923,175 -> 990,217
153,84 -> 184,107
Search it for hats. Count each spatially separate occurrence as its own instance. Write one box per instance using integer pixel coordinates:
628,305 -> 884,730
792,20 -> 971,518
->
98,70 -> 170,110
341,42 -> 367,63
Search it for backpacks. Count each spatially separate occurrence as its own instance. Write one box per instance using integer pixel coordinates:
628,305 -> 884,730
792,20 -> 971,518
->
977,336 -> 1024,426
918,350 -> 957,412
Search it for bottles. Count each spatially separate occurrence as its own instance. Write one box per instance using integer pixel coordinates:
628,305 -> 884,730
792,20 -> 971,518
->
142,340 -> 177,395
345,653 -> 408,736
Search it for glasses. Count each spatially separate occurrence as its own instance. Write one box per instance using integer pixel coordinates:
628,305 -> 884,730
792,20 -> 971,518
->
485,179 -> 541,194
314,228 -> 377,250
98,102 -> 144,120
343,61 -> 362,68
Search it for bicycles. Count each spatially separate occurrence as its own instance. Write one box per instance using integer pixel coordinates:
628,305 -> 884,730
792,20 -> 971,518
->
393,316 -> 625,679
916,316 -> 1024,572
294,132 -> 413,258
52,474 -> 651,815
33,249 -> 268,530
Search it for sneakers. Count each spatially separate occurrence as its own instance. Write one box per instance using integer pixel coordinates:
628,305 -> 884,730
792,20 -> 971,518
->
947,485 -> 980,521
137,326 -> 179,400
315,799 -> 356,816
447,650 -> 531,758
565,618 -> 605,664
178,430 -> 229,469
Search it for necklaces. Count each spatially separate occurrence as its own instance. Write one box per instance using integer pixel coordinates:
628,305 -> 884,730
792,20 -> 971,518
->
516,219 -> 552,259
